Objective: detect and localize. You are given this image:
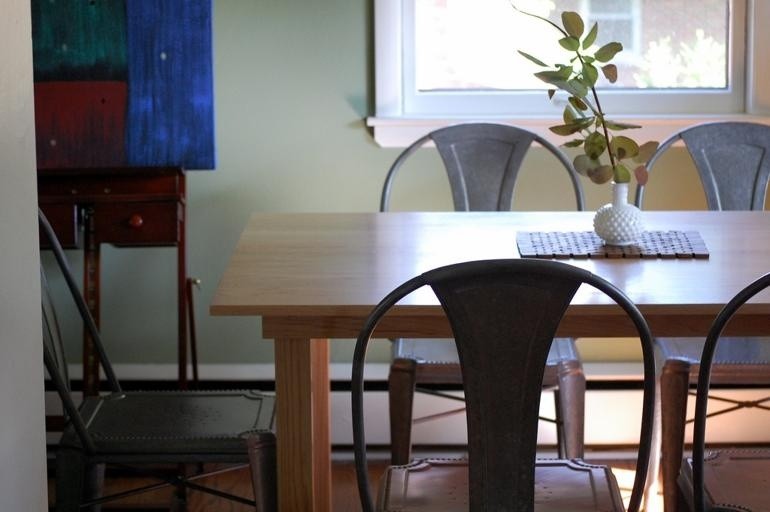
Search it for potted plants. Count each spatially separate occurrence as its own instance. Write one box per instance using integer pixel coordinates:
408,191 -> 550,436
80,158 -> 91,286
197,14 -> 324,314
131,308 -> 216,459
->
522,5 -> 661,245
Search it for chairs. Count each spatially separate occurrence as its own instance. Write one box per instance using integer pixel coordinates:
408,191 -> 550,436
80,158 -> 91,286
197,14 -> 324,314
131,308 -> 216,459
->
41,212 -> 279,510
380,123 -> 586,459
636,120 -> 770,512
674,274 -> 770,509
351,258 -> 655,512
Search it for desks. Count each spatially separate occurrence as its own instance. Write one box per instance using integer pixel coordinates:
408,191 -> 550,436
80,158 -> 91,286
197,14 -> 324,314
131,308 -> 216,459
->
40,162 -> 205,393
210,210 -> 769,512
